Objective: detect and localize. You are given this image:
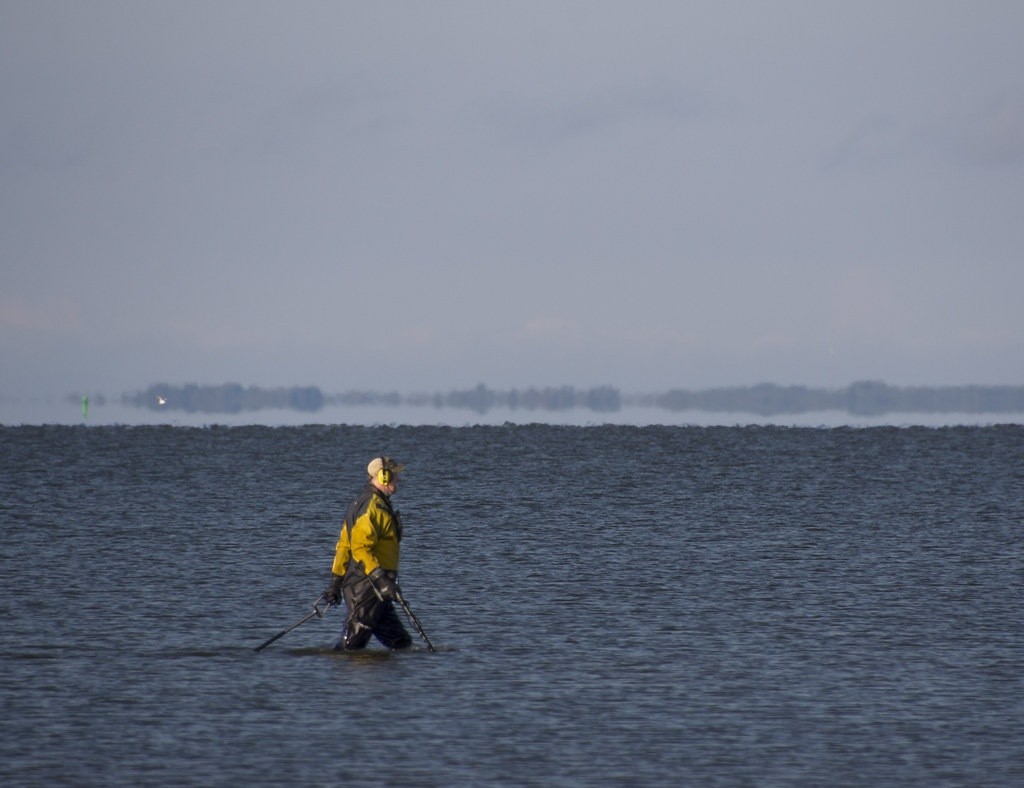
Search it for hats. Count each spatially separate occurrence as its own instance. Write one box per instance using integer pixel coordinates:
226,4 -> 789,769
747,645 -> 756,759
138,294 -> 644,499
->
367,456 -> 405,476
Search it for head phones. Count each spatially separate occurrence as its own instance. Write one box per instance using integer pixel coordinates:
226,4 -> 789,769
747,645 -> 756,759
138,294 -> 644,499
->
378,457 -> 392,486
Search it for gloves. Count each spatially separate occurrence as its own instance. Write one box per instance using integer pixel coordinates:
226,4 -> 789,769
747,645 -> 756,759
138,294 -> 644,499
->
376,574 -> 401,603
321,574 -> 343,608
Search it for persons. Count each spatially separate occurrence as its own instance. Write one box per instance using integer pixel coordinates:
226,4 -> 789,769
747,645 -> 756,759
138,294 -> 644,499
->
321,456 -> 414,653
